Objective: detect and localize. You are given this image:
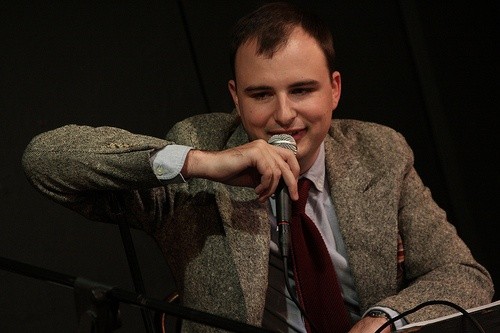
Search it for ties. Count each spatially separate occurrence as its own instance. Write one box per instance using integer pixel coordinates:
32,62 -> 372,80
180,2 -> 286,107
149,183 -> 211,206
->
288,176 -> 353,333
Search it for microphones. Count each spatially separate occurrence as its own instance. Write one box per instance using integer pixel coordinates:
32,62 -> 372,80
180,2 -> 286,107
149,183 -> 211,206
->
267,134 -> 298,257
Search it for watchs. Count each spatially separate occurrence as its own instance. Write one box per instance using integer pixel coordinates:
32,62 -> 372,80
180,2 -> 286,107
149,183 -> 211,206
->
367,309 -> 395,332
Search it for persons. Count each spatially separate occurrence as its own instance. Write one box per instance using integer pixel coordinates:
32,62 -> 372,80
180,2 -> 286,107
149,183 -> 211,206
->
22,7 -> 495,333
